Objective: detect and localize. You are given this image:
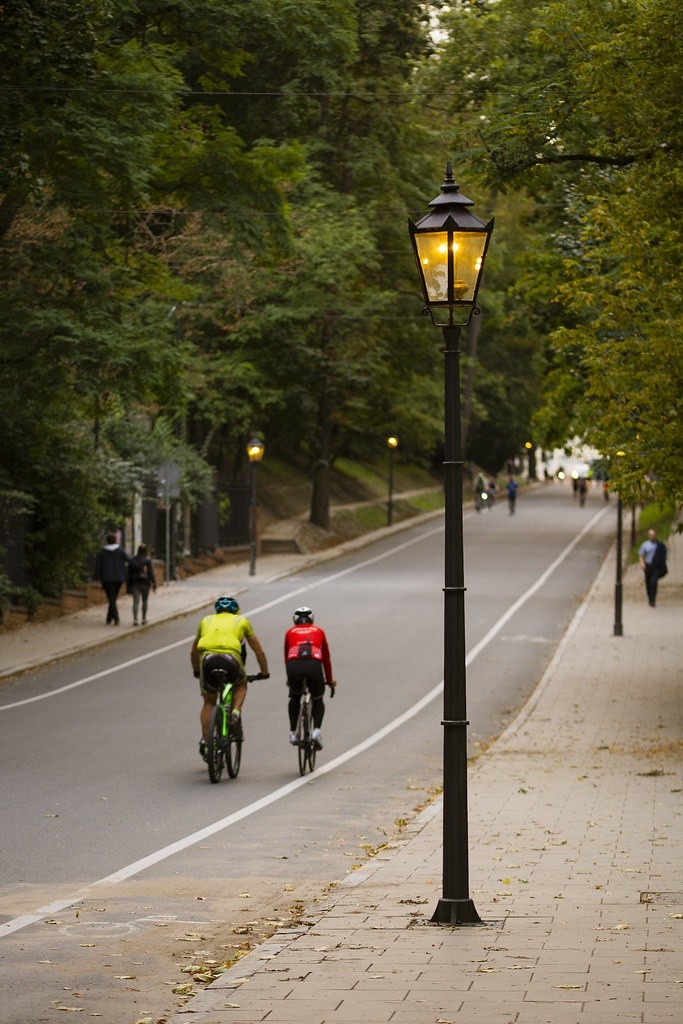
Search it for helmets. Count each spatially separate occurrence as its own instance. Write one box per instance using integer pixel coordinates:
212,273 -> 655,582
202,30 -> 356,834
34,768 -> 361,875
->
214,596 -> 239,613
292,607 -> 314,626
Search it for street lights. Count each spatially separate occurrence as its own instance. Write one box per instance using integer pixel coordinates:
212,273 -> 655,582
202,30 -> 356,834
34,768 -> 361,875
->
244,435 -> 266,578
386,434 -> 400,525
406,157 -> 495,923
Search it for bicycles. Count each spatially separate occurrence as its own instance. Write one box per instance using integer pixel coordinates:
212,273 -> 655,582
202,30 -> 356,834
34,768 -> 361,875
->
194,674 -> 267,783
287,682 -> 334,777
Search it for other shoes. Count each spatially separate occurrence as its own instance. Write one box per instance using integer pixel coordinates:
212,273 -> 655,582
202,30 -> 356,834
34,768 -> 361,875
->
199,739 -> 208,758
311,729 -> 323,752
229,713 -> 244,740
289,732 -> 298,746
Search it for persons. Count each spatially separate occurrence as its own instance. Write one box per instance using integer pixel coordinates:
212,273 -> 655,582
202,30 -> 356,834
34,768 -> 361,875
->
505,478 -> 519,515
596,469 -> 610,494
126,543 -> 157,626
284,607 -> 336,751
471,471 -> 487,506
573,471 -> 589,498
638,529 -> 666,607
487,476 -> 499,500
94,534 -> 132,625
191,596 -> 268,753
